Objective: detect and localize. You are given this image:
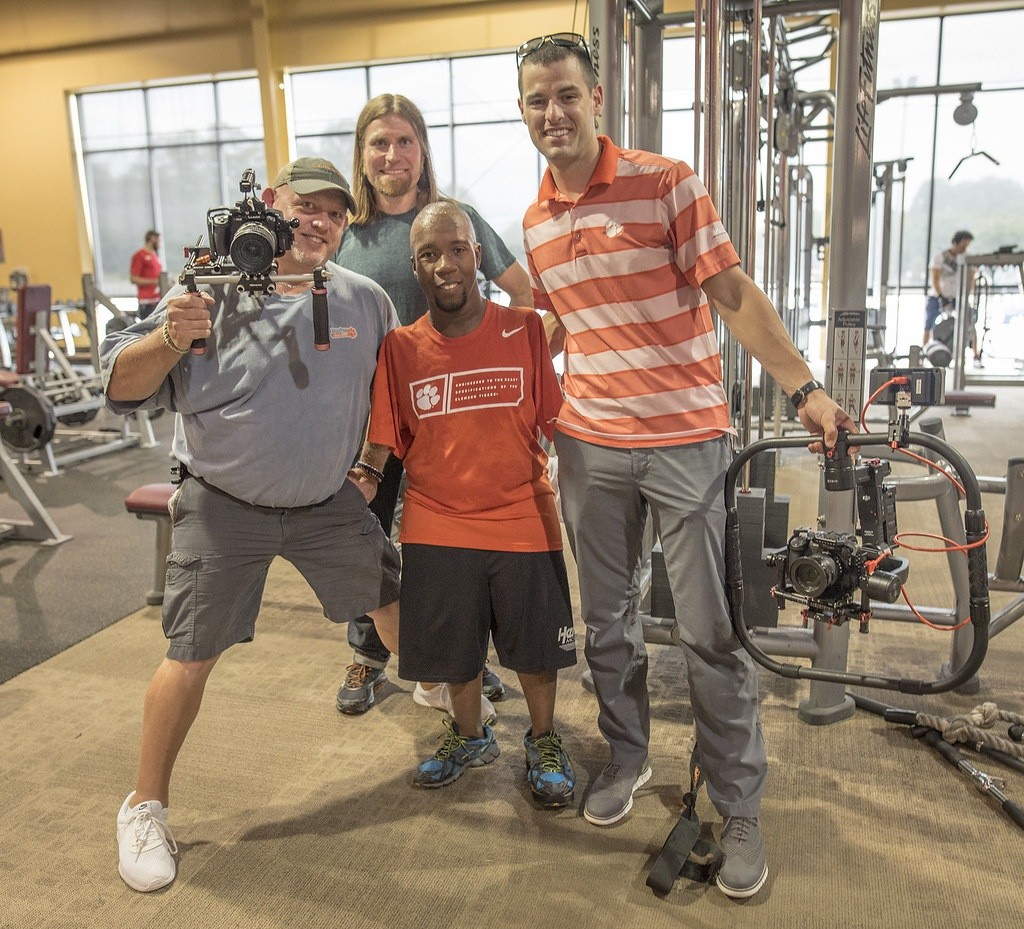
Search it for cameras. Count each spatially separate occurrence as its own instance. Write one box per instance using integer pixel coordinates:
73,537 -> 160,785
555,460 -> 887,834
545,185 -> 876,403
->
788,529 -> 865,600
205,197 -> 300,276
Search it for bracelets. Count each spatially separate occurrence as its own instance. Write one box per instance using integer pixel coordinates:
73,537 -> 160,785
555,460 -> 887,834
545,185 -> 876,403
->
789,380 -> 824,409
355,459 -> 384,484
161,322 -> 191,355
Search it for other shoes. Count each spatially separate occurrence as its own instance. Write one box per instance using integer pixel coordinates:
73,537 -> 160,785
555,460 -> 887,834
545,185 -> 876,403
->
973,355 -> 984,368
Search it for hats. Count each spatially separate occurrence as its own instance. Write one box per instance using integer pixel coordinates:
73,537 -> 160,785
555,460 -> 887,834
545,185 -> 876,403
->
273,156 -> 359,218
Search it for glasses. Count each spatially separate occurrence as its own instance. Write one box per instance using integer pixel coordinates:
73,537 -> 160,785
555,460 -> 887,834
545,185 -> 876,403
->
516,32 -> 594,74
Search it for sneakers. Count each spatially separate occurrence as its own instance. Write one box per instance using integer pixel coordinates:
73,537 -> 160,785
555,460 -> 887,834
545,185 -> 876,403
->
583,760 -> 652,825
411,718 -> 500,787
715,815 -> 768,897
481,660 -> 505,699
523,724 -> 576,807
335,661 -> 386,714
413,679 -> 496,726
116,791 -> 178,893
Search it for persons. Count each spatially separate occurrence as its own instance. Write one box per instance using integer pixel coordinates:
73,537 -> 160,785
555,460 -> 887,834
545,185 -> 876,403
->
368,199 -> 579,810
334,92 -> 533,715
514,30 -> 860,901
99,158 -> 496,893
921,230 -> 984,367
130,230 -> 164,321
